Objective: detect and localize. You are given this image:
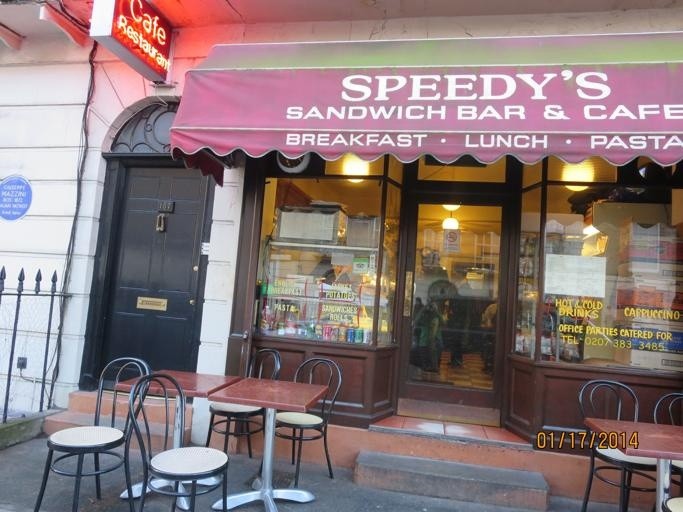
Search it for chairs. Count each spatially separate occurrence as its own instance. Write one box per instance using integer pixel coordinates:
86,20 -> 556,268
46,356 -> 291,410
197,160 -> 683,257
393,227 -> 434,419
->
130,372 -> 228,511
653,392 -> 683,511
205,346 -> 280,455
259,358 -> 343,489
32,356 -> 151,511
577,380 -> 672,512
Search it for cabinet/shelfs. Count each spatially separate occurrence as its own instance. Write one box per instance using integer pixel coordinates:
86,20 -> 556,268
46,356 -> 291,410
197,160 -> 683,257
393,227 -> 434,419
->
264,241 -> 388,342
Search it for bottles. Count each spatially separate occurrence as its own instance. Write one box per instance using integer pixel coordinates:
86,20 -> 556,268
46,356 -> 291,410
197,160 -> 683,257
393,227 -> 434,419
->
263,304 -> 271,322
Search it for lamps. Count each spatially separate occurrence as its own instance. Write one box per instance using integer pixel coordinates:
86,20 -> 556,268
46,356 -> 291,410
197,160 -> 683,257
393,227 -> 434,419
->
442,204 -> 460,230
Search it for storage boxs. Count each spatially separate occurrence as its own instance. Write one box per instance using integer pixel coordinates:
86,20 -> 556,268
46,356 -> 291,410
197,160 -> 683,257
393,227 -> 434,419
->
618,222 -> 683,261
614,304 -> 683,371
615,260 -> 683,311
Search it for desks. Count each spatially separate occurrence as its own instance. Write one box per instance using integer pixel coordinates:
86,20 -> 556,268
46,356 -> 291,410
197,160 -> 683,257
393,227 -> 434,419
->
583,418 -> 683,511
208,378 -> 329,510
114,368 -> 243,509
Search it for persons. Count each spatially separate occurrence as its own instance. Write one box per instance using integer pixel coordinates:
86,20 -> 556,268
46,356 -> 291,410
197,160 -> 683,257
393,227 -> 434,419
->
412,297 -> 496,373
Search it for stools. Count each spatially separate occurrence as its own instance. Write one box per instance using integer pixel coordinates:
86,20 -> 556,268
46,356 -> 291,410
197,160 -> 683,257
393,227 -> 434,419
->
663,495 -> 683,510
